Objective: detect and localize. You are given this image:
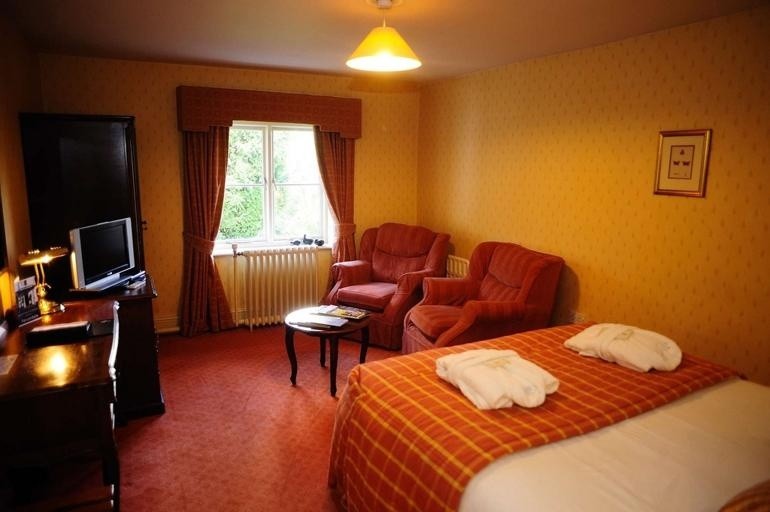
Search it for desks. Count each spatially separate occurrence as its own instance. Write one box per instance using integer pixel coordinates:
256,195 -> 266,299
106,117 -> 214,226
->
54,269 -> 165,427
0,299 -> 122,512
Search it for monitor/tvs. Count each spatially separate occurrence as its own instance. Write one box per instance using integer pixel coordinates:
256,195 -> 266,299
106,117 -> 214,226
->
69,217 -> 136,290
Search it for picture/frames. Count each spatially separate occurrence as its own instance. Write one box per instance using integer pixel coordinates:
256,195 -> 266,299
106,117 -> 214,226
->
652,127 -> 713,199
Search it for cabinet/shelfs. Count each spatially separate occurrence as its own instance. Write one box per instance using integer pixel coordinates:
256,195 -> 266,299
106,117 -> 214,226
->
17,108 -> 147,272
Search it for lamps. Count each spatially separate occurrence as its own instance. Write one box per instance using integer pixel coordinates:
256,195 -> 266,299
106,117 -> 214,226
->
18,245 -> 70,315
344,0 -> 423,77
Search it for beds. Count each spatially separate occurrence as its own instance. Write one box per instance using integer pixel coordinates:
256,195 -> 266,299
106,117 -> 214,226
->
326,323 -> 770,512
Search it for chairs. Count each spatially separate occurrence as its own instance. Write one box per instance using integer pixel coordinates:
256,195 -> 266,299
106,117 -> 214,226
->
401,240 -> 565,356
314,222 -> 451,350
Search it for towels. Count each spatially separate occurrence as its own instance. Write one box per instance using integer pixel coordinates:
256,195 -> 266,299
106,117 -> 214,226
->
435,348 -> 560,410
564,324 -> 683,373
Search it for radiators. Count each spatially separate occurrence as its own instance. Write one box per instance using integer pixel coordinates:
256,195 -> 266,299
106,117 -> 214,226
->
241,247 -> 320,332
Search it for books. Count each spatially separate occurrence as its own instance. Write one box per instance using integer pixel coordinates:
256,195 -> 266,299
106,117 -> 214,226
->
288,304 -> 373,329
